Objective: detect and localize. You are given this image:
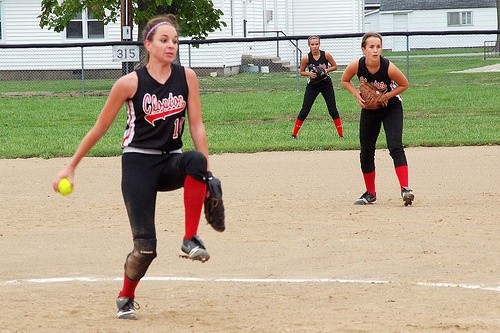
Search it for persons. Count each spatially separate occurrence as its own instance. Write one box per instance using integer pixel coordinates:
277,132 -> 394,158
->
341,32 -> 415,206
53,15 -> 221,318
291,34 -> 344,138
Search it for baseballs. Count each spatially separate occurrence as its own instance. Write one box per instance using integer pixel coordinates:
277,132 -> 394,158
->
57,178 -> 72,194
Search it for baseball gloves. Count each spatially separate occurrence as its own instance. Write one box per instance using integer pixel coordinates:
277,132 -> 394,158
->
311,65 -> 331,85
359,81 -> 387,110
203,170 -> 226,232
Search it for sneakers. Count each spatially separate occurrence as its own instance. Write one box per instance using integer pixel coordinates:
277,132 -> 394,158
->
115,297 -> 140,319
179,234 -> 210,263
401,187 -> 414,206
356,191 -> 376,205
